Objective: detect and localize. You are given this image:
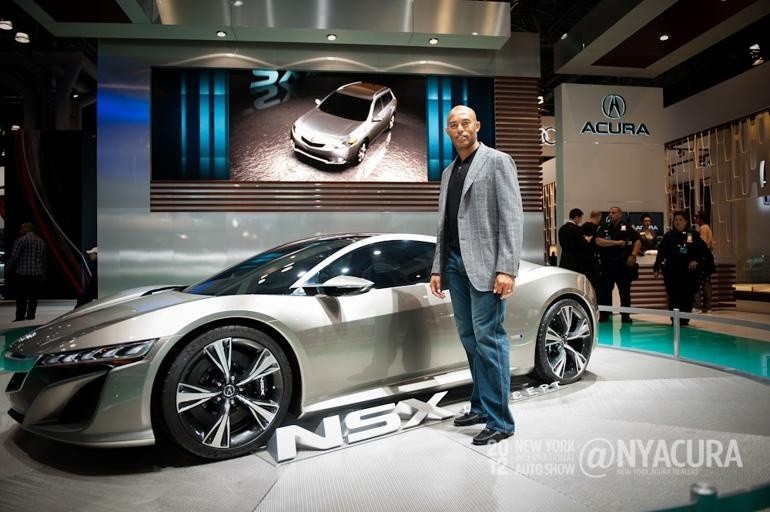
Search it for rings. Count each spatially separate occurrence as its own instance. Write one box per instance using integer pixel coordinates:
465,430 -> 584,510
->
508,289 -> 511,292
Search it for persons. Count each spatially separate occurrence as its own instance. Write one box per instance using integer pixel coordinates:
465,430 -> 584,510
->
83,247 -> 97,299
428,105 -> 524,445
554,205 -> 718,327
7,222 -> 50,322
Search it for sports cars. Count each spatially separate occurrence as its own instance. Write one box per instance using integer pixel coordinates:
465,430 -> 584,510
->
0,228 -> 602,468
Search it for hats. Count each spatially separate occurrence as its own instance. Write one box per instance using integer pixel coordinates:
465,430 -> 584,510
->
86,247 -> 97,254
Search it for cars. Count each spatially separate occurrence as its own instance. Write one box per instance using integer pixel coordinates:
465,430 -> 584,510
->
285,80 -> 399,172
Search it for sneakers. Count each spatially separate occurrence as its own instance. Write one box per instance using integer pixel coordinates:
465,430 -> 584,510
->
692,307 -> 712,314
13,317 -> 35,322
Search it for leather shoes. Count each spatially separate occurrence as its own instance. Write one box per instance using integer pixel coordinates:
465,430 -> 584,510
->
600,312 -> 632,322
473,427 -> 514,444
454,412 -> 487,425
671,318 -> 689,325
